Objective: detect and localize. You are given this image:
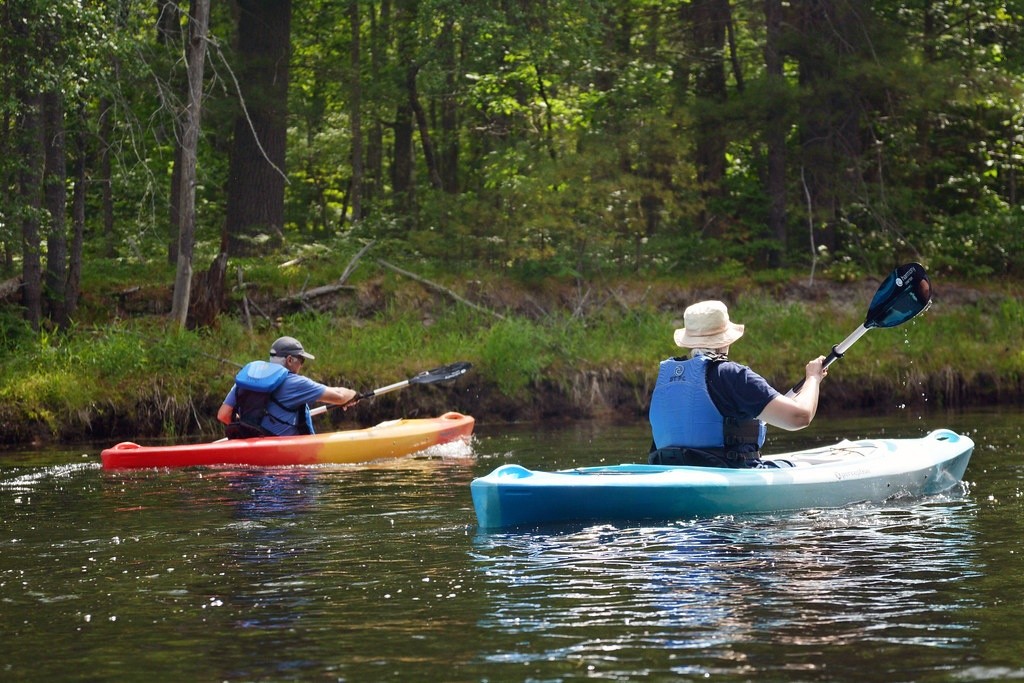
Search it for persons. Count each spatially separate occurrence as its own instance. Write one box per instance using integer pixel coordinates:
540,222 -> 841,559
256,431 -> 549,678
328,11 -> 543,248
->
217,336 -> 360,441
648,300 -> 828,469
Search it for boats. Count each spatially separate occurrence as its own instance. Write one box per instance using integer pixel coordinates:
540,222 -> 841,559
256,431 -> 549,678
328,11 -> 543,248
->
469,428 -> 974,534
101,412 -> 475,473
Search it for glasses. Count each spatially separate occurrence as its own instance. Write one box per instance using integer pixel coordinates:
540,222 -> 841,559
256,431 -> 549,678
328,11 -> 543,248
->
293,356 -> 306,364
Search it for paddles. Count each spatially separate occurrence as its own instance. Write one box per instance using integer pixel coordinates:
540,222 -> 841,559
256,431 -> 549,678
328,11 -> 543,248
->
758,261 -> 932,429
214,361 -> 475,442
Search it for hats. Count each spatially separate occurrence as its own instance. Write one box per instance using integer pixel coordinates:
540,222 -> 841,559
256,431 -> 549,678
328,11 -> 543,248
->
673,300 -> 746,350
268,335 -> 316,361
